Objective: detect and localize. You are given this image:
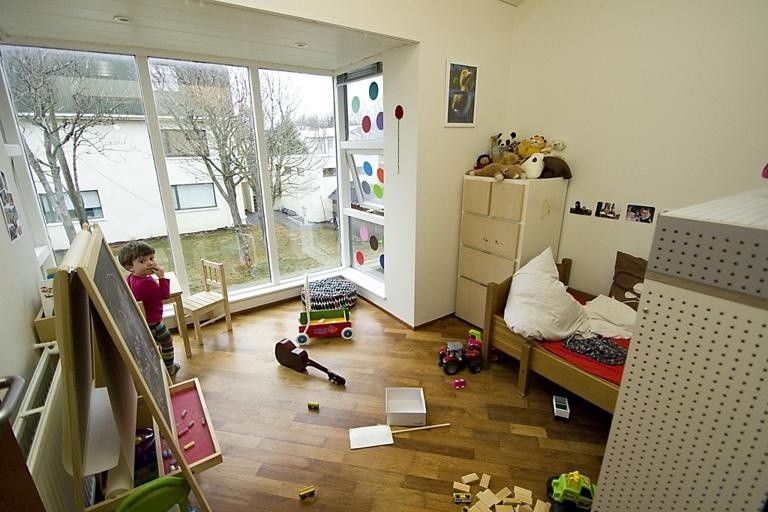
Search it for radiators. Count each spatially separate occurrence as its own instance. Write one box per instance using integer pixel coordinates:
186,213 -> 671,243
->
11,341 -> 96,512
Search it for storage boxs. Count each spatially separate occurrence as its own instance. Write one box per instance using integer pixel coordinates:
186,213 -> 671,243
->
385,387 -> 426,426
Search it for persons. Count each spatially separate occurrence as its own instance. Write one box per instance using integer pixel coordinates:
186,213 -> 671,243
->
118,240 -> 180,376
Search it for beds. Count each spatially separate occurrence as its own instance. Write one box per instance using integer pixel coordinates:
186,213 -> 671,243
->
481,258 -> 636,415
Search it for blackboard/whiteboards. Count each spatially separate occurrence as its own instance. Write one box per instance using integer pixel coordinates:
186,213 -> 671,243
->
78,223 -> 178,447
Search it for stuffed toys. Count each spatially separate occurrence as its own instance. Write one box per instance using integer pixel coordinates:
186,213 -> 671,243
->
467,131 -> 572,181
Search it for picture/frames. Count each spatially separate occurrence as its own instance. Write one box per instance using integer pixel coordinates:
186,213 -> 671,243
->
444,58 -> 479,128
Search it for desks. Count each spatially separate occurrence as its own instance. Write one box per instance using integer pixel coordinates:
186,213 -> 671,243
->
152,272 -> 192,358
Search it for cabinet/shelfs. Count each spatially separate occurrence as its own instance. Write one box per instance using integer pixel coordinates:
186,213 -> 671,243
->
455,175 -> 569,329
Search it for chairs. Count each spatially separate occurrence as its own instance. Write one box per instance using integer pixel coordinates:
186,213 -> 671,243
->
173,258 -> 233,346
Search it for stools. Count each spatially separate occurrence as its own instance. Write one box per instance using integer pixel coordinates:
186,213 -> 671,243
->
301,279 -> 357,310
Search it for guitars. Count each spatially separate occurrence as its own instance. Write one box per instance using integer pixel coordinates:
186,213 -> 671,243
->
275,339 -> 345,386
